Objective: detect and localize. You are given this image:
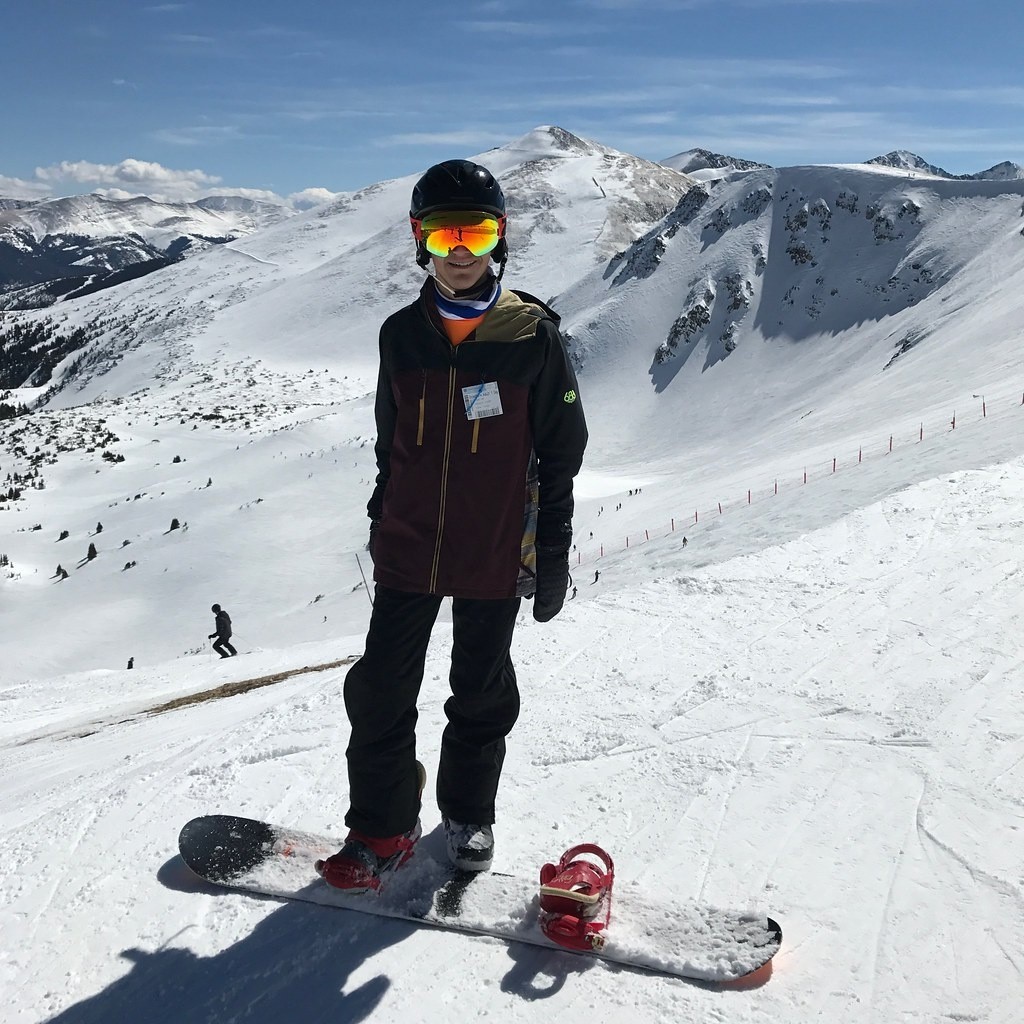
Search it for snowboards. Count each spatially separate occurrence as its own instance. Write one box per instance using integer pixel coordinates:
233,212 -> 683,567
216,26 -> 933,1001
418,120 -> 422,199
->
179,813 -> 779,984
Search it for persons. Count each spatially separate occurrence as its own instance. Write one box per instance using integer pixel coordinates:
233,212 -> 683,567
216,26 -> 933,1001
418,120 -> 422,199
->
595,570 -> 601,581
127,656 -> 134,669
683,537 -> 688,547
208,604 -> 238,660
588,488 -> 642,539
324,159 -> 589,893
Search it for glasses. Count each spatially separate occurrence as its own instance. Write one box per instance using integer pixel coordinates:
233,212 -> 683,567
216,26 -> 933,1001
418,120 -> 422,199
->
421,210 -> 499,258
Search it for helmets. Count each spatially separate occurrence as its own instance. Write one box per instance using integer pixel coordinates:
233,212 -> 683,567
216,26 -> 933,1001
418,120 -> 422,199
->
409,159 -> 507,264
212,604 -> 221,613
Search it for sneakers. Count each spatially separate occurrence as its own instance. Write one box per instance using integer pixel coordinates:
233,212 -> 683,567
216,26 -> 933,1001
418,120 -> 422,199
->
322,817 -> 423,895
441,809 -> 494,872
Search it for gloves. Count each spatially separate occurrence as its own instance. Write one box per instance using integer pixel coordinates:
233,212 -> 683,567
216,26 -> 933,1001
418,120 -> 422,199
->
522,549 -> 569,623
208,635 -> 215,639
